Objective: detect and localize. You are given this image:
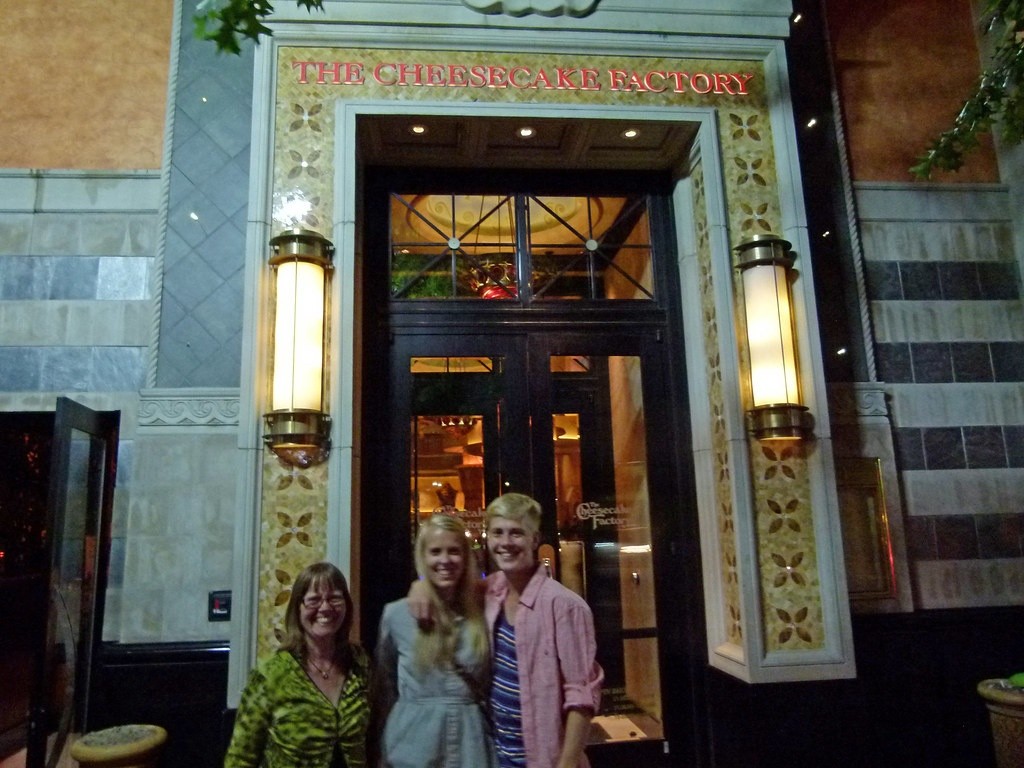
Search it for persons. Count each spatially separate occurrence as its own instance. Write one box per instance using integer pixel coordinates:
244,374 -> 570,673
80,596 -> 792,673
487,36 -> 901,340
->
475,492 -> 605,768
224,564 -> 386,768
373,515 -> 500,768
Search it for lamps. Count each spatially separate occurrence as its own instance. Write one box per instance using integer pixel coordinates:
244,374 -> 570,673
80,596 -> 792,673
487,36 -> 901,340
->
263,228 -> 338,471
425,415 -> 478,440
459,195 -> 519,300
731,231 -> 810,447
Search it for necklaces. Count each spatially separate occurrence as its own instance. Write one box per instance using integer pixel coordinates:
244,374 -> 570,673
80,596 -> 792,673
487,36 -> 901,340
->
307,657 -> 335,679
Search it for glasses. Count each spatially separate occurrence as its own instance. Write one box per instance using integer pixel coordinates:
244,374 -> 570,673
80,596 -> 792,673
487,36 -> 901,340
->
302,594 -> 346,606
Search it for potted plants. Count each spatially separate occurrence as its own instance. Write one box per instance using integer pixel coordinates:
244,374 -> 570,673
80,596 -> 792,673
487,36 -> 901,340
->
976,673 -> 1024,768
